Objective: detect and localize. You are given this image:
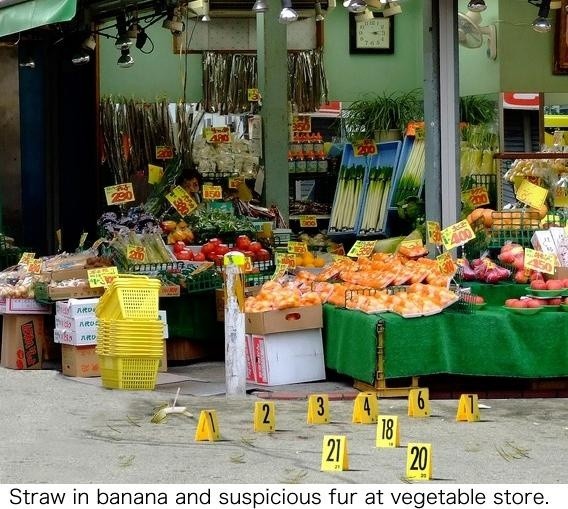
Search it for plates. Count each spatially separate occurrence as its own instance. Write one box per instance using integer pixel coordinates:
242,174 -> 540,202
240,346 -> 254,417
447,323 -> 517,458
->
459,287 -> 568,315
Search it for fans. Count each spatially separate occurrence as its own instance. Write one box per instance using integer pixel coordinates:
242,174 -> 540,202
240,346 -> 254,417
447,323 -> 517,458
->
458,10 -> 497,61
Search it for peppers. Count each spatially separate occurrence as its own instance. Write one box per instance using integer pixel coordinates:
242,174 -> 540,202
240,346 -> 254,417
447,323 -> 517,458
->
193,208 -> 257,234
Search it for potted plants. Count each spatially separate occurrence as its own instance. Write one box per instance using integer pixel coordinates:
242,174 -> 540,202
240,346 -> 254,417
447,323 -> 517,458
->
327,87 -> 496,144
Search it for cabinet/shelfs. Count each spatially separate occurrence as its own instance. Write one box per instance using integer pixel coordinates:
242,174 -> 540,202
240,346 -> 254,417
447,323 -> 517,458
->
193,163 -> 331,221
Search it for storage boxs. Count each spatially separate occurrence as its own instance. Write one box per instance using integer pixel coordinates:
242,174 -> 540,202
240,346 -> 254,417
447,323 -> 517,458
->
327,134 -> 424,251
215,286 -> 326,386
530,226 -> 568,280
0,265 -> 168,377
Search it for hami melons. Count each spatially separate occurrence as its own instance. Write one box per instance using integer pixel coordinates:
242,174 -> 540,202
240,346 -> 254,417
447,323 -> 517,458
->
466,204 -> 548,240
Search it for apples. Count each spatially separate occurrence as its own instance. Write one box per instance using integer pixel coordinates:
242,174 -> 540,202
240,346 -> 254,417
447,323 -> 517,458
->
462,243 -> 568,307
173,235 -> 270,266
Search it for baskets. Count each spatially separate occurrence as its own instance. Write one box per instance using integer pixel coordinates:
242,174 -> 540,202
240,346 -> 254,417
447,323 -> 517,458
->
95,273 -> 164,391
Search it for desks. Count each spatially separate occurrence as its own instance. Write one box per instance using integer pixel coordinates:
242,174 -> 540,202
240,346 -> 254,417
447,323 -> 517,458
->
323,279 -> 568,400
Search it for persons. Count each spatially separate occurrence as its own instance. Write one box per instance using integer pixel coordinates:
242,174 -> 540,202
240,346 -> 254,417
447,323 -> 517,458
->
174,167 -> 207,201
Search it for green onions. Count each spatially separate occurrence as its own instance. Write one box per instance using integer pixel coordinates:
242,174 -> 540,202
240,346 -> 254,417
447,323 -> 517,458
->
330,139 -> 426,235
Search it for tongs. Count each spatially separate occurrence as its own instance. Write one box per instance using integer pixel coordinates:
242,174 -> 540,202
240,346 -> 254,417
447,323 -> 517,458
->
99,50 -> 330,196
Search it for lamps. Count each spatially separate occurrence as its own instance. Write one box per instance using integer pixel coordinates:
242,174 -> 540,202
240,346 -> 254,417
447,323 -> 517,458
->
467,0 -> 487,13
527,0 -> 553,34
17,0 -> 402,68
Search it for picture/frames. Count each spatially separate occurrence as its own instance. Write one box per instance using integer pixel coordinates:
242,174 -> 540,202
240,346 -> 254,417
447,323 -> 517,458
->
553,0 -> 568,75
